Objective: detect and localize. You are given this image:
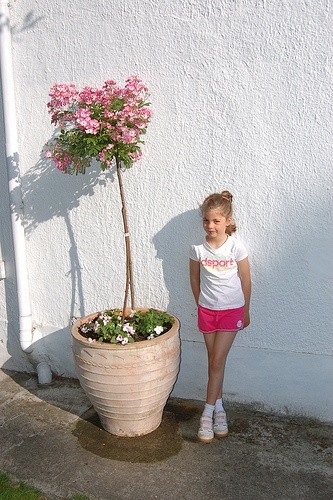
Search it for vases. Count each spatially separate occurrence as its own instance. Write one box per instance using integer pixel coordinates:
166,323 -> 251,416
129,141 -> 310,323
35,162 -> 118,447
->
70,308 -> 181,438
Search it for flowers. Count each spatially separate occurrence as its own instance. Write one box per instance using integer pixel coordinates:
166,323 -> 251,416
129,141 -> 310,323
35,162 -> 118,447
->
43,76 -> 175,346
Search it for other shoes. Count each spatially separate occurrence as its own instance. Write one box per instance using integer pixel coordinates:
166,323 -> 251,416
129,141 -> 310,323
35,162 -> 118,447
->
197,414 -> 213,443
213,411 -> 228,437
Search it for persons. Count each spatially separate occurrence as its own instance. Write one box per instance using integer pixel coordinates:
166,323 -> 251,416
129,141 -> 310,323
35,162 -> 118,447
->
188,191 -> 251,443
